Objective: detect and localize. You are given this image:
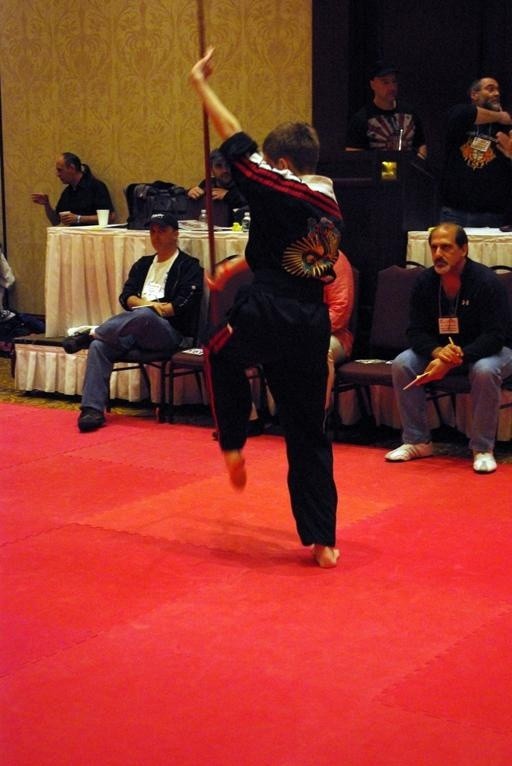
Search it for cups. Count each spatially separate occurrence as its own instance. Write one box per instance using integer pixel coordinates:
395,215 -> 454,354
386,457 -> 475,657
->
59,211 -> 71,226
96,208 -> 109,228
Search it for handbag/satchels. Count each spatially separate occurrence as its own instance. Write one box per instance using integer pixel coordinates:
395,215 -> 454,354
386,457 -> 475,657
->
126,181 -> 187,230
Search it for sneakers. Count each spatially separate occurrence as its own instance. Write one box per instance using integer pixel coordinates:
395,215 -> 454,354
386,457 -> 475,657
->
63,328 -> 92,354
384,441 -> 433,461
473,452 -> 497,472
78,410 -> 105,431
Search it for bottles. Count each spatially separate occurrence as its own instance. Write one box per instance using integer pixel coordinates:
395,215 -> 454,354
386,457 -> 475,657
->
240,212 -> 251,233
199,209 -> 209,230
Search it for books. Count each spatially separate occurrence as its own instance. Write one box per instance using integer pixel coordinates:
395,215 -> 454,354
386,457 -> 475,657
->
131,303 -> 164,318
402,370 -> 432,393
176,219 -> 221,233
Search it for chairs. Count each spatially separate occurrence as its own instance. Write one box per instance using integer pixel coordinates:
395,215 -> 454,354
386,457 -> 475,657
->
334,257 -> 438,447
421,263 -> 512,444
261,265 -> 375,439
106,266 -> 203,425
169,253 -> 250,433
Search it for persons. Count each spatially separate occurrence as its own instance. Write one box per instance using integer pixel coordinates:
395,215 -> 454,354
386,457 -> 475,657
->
384,220 -> 511,474
190,42 -> 344,569
29,152 -> 117,226
438,76 -> 511,226
323,249 -> 356,425
186,147 -> 249,228
61,209 -> 204,431
341,51 -> 429,161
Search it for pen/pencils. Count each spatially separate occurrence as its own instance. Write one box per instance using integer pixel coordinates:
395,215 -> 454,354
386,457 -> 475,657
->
447,336 -> 456,345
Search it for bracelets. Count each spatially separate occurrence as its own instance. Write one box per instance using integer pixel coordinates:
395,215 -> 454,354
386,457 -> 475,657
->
77,215 -> 81,222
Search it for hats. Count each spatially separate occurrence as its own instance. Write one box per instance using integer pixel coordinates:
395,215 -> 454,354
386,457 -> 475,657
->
367,61 -> 401,77
145,212 -> 178,229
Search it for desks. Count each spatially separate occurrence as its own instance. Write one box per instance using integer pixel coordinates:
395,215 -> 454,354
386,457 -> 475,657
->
43,219 -> 512,354
